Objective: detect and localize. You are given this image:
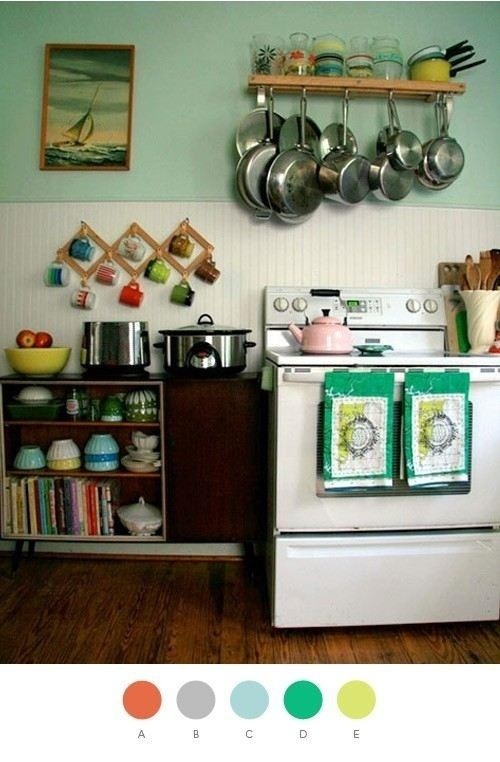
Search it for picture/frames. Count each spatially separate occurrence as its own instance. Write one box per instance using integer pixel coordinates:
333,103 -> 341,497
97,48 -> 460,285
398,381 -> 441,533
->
39,44 -> 135,169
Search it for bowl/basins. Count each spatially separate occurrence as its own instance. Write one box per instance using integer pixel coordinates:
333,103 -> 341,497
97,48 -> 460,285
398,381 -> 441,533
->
100,388 -> 158,423
283,32 -> 405,81
131,431 -> 160,453
3,346 -> 73,378
12,432 -> 120,473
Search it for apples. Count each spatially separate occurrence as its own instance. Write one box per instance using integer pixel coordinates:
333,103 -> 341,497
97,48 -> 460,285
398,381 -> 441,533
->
35,332 -> 53,347
16,329 -> 35,348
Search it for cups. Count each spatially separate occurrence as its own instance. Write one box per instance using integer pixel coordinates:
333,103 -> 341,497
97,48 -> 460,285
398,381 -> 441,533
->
117,234 -> 147,264
145,258 -> 170,284
252,31 -> 283,77
68,278 -> 96,308
193,255 -> 221,283
67,234 -> 95,264
93,259 -> 120,286
458,289 -> 500,354
44,260 -> 71,287
169,279 -> 195,306
119,279 -> 144,308
168,234 -> 195,259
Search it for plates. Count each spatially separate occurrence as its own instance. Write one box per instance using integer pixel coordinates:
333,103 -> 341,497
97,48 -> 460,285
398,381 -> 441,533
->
13,386 -> 60,405
119,445 -> 161,474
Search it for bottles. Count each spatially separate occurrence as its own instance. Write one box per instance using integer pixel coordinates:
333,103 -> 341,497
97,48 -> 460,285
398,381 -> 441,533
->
66,387 -> 82,422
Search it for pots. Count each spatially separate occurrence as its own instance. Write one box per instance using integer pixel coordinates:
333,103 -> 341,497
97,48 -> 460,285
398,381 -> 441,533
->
81,320 -> 152,380
234,85 -> 465,226
407,39 -> 487,84
151,312 -> 257,379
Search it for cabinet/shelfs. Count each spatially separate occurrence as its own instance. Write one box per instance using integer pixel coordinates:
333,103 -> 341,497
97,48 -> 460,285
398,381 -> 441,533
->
0,377 -> 166,543
164,381 -> 268,543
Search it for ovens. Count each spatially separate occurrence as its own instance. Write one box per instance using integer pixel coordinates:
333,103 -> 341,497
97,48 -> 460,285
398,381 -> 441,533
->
264,359 -> 499,629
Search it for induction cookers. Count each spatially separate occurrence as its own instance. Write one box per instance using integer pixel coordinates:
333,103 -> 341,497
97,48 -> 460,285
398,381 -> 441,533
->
272,346 -> 500,357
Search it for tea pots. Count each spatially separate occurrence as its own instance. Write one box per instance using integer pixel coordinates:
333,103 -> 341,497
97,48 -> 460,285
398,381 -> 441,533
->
288,308 -> 354,355
